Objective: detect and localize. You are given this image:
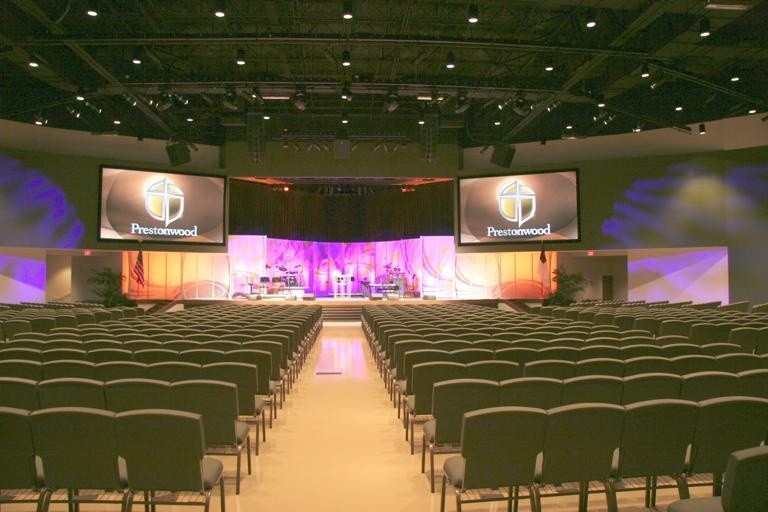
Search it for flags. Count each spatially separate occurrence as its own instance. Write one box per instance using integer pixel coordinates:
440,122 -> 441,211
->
536,251 -> 550,289
133,252 -> 145,287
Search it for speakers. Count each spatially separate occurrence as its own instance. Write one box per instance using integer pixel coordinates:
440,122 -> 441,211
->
165,143 -> 191,166
491,145 -> 515,168
602,275 -> 613,301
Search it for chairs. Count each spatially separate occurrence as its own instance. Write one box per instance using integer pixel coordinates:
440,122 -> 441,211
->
361,300 -> 768,512
0,301 -> 322,512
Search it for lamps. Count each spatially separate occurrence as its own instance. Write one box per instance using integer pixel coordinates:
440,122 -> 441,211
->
416,1 -> 480,127
746,103 -> 757,114
699,17 -> 712,39
67,91 -> 83,119
595,93 -> 607,110
728,64 -> 740,83
673,102 -> 683,112
338,2 -> 355,128
84,4 -> 195,128
214,1 -> 272,123
34,113 -> 48,127
27,53 -> 39,70
640,64 -> 649,79
631,120 -> 642,133
592,111 -> 618,126
494,13 -> 597,136
